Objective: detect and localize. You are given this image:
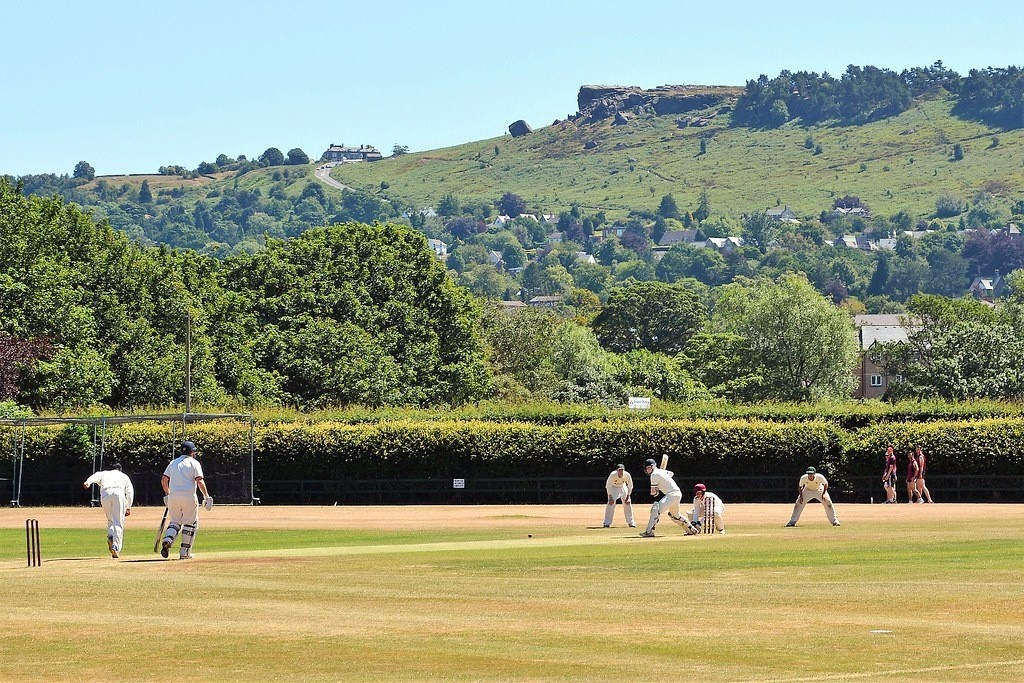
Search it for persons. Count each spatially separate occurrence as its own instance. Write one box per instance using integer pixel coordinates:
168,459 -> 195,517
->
639,459 -> 698,536
784,466 -> 842,527
684,484 -> 725,535
882,446 -> 897,504
84,461 -> 134,558
161,441 -> 213,559
603,464 -> 636,527
905,451 -> 924,504
916,445 -> 934,504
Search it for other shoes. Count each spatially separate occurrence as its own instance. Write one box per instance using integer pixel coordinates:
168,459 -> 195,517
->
833,522 -> 840,526
883,498 -> 897,504
684,528 -> 699,536
161,541 -> 169,558
639,532 -> 654,537
908,500 -> 913,504
629,524 -> 635,527
916,498 -> 924,503
719,529 -> 724,534
111,546 -> 119,558
108,538 -> 113,552
784,522 -> 795,527
180,547 -> 194,559
604,524 -> 609,527
928,500 -> 934,503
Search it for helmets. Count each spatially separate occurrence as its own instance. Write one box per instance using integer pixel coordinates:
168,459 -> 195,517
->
644,459 -> 656,467
695,484 -> 706,492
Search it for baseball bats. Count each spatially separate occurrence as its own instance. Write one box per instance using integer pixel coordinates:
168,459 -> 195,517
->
154,507 -> 169,552
660,454 -> 668,470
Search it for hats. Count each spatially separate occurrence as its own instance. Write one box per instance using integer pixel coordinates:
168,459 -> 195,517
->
616,464 -> 625,469
181,441 -> 197,452
806,466 -> 816,473
887,447 -> 893,451
